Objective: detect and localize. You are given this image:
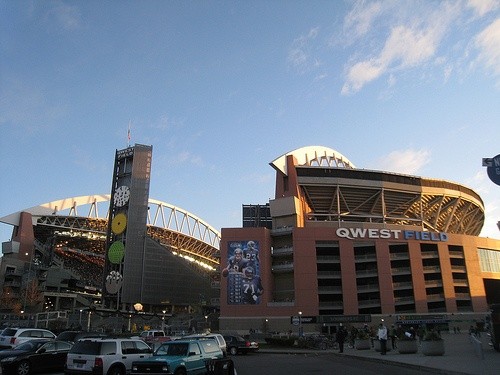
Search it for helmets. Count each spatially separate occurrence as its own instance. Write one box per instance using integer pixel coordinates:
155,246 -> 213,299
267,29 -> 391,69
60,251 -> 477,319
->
233,248 -> 243,263
244,267 -> 254,279
246,241 -> 255,249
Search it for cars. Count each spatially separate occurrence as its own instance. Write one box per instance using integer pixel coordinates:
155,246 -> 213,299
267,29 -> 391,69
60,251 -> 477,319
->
223,335 -> 260,356
0,327 -> 74,375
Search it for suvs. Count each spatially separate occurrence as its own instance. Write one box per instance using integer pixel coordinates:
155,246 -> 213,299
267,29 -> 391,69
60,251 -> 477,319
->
55,331 -> 154,375
130,330 -> 228,375
314,335 -> 330,340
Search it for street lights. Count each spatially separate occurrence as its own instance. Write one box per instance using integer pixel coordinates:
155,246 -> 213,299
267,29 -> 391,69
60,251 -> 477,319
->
205,315 -> 208,333
79,310 -> 82,329
381,317 -> 384,325
45,297 -> 52,313
162,309 -> 166,331
298,312 -> 302,340
20,310 -> 24,328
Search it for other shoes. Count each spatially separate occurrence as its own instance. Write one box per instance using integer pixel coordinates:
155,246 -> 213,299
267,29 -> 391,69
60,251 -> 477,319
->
381,352 -> 386,355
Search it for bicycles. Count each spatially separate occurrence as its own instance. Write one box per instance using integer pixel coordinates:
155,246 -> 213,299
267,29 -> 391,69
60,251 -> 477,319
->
320,340 -> 340,351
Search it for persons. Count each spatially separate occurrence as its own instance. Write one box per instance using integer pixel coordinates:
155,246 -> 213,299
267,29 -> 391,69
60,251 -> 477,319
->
438,328 -> 441,337
336,326 -> 348,354
409,326 -> 416,341
189,316 -> 195,332
351,326 -> 358,348
363,324 -> 374,347
71,321 -> 81,330
377,323 -> 388,355
390,324 -> 405,349
418,321 -> 425,345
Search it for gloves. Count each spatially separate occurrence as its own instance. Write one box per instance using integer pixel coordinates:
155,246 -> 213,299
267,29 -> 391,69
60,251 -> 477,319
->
377,336 -> 379,340
381,338 -> 384,342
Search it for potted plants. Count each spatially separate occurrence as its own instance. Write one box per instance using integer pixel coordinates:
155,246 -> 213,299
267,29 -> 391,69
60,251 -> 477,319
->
374,328 -> 392,351
421,329 -> 445,355
397,327 -> 418,354
354,330 -> 371,349
